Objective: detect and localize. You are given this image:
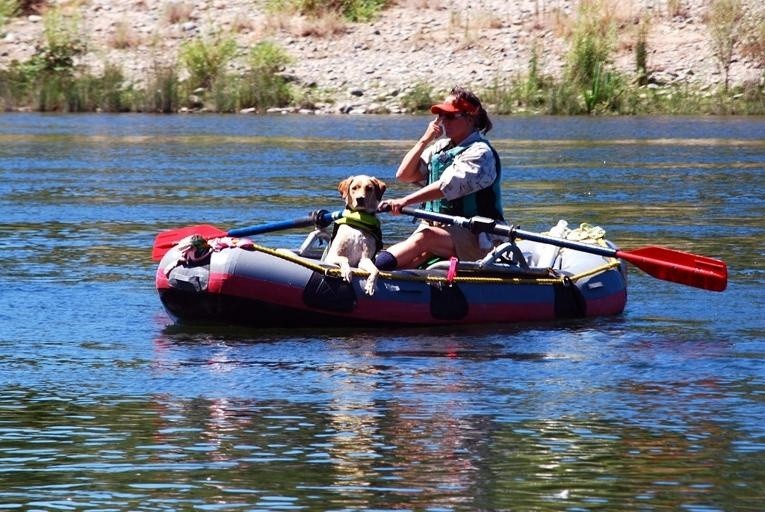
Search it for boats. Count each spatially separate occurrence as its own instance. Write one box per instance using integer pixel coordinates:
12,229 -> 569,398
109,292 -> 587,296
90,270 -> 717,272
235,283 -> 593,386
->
156,228 -> 628,330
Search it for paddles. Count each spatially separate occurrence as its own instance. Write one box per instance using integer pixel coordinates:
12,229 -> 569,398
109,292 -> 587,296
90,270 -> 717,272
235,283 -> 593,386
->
399,206 -> 728,292
151,204 -> 391,262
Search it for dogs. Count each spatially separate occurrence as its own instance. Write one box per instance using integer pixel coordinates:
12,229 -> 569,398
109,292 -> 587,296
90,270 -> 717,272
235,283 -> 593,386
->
323,174 -> 388,297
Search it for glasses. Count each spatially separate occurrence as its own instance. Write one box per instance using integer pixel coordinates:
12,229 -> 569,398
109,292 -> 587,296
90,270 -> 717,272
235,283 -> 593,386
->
438,111 -> 473,120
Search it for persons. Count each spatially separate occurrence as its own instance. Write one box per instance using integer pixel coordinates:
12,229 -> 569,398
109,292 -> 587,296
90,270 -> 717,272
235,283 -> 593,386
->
374,90 -> 506,269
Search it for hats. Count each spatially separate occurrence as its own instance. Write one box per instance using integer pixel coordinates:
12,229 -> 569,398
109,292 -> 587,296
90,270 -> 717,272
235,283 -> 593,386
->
431,94 -> 481,117
